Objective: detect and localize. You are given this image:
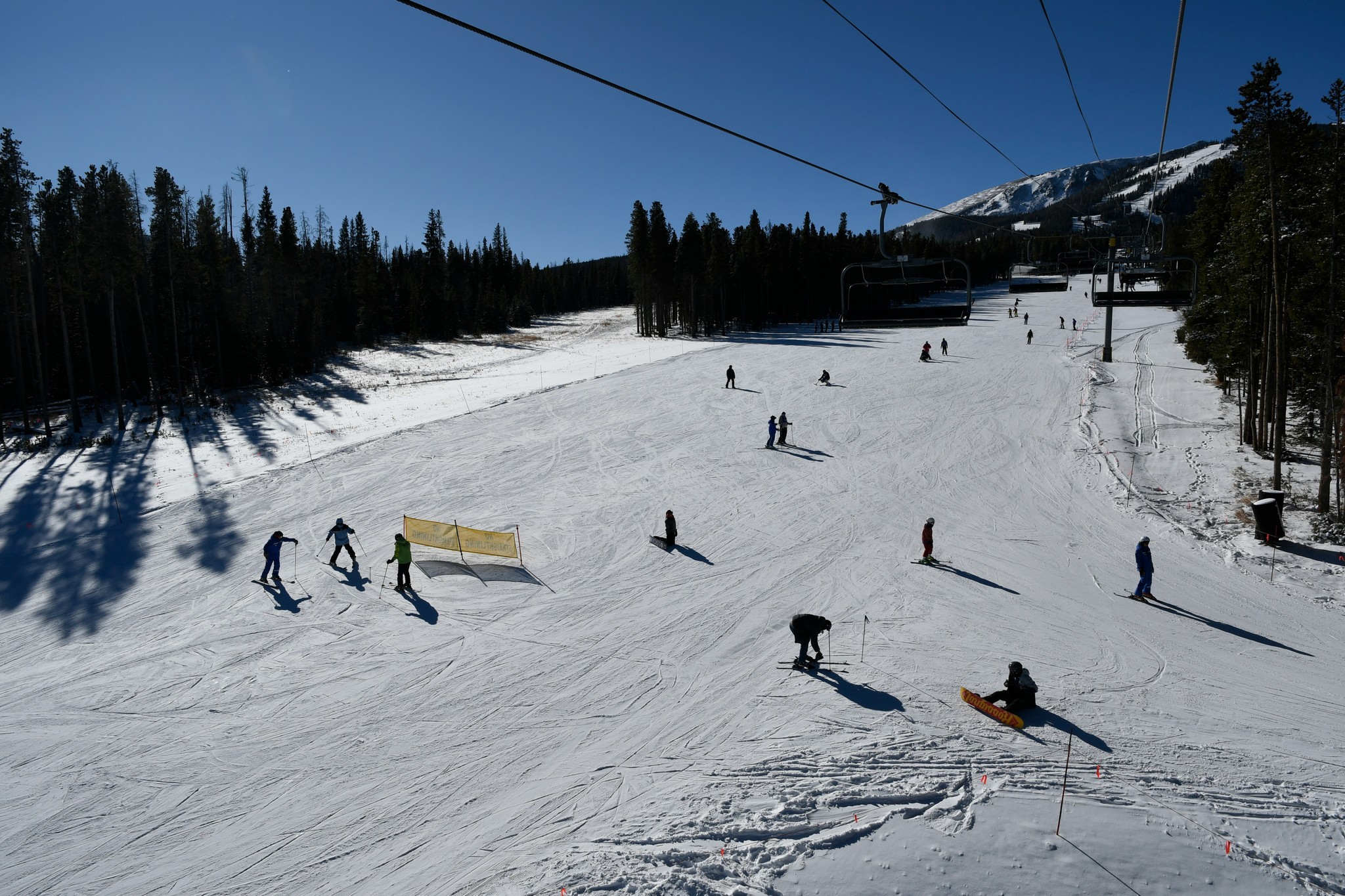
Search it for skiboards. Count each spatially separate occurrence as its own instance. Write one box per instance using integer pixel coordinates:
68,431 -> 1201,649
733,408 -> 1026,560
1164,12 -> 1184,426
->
816,381 -> 835,386
250,575 -> 296,588
381,582 -> 422,595
1113,588 -> 1162,605
755,446 -> 790,450
943,354 -> 948,356
773,442 -> 796,446
321,554 -> 357,569
776,660 -> 847,672
721,387 -> 740,390
910,559 -> 954,567
1026,343 -> 1031,345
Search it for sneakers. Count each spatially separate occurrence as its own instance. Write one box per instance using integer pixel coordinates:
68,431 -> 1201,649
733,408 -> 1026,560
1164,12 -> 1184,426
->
922,556 -> 934,563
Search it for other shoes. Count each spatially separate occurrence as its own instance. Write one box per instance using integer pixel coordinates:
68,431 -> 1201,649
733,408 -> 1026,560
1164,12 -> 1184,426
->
1135,592 -> 1153,601
352,557 -> 356,560
396,583 -> 411,590
767,441 -> 787,448
799,655 -> 813,668
260,575 -> 280,583
330,560 -> 336,564
726,386 -> 735,389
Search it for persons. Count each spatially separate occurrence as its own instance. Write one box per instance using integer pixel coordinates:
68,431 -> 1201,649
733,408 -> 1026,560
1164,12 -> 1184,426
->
387,533 -> 412,590
921,517 -> 936,564
260,531 -> 298,583
818,370 -> 830,383
812,319 -> 843,333
970,249 -> 1155,330
940,338 -> 948,355
778,412 -> 793,445
790,614 -> 832,668
764,416 -> 779,448
919,349 -> 931,362
326,518 -> 356,564
973,661 -> 1039,719
923,341 -> 931,355
1026,329 -> 1033,344
726,365 -> 736,389
653,509 -> 677,548
1134,535 -> 1154,601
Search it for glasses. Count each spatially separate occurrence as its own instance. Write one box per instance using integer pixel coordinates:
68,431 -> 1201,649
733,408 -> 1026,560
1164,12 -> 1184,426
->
1144,542 -> 1149,544
669,512 -> 673,515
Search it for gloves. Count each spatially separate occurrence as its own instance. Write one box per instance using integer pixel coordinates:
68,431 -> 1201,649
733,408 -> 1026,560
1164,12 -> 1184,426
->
351,529 -> 355,534
326,536 -> 330,541
789,422 -> 792,425
816,653 -> 823,661
1139,567 -> 1144,576
1152,563 -> 1154,573
266,557 -> 268,562
295,540 -> 298,545
929,540 -> 933,545
675,531 -> 677,537
386,560 -> 394,564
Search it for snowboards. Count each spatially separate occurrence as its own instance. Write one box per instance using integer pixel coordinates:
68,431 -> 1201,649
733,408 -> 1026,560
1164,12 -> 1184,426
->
959,685 -> 1024,730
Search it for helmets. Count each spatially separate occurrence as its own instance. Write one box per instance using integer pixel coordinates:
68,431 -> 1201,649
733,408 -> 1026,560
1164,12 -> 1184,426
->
273,531 -> 283,540
1009,661 -> 1023,676
770,412 -> 785,420
926,517 -> 935,525
1140,536 -> 1150,543
395,533 -> 402,540
336,518 -> 343,527
